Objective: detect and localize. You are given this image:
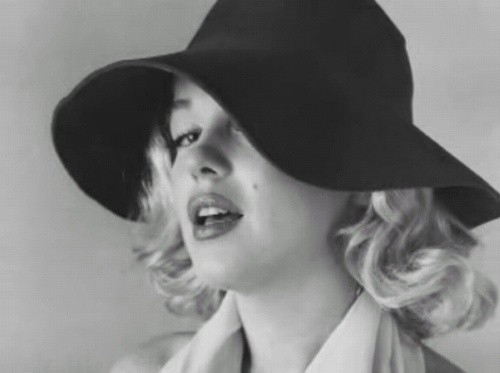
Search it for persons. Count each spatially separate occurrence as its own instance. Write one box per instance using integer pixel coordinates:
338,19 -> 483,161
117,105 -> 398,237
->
49,0 -> 500,373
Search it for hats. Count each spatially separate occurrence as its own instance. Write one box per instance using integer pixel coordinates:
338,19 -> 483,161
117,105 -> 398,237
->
49,0 -> 500,233
